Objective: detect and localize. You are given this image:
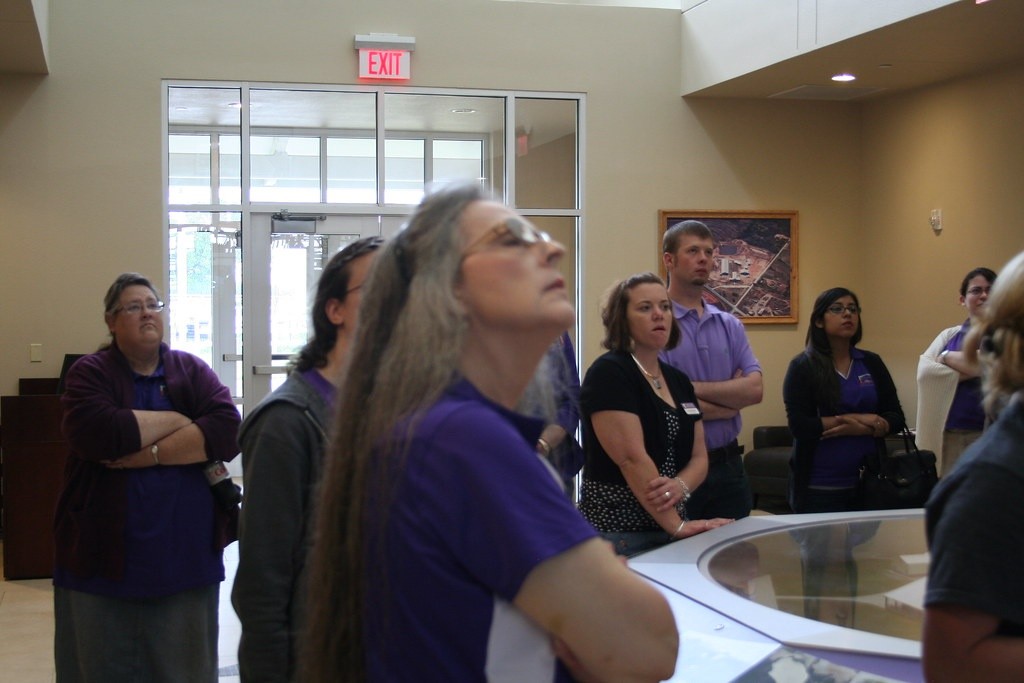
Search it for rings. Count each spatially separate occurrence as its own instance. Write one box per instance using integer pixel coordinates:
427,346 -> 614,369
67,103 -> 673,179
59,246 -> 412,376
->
878,422 -> 881,425
665,491 -> 672,500
704,522 -> 709,526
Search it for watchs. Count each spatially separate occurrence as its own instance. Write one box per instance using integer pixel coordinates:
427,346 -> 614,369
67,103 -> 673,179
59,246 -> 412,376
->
940,350 -> 952,367
150,442 -> 160,465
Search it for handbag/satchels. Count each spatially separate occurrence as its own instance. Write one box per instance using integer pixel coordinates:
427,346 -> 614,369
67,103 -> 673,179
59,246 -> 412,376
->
866,413 -> 939,509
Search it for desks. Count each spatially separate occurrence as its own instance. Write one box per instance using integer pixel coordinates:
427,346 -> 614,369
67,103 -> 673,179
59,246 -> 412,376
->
624,508 -> 930,683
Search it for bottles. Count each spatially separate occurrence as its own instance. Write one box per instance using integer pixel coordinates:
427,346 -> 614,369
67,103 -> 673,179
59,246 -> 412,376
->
200,458 -> 242,511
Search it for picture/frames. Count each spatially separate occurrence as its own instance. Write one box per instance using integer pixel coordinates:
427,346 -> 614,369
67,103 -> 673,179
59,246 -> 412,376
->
658,209 -> 800,325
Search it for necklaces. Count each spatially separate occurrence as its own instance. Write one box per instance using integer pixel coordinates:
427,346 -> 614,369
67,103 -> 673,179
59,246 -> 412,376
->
631,352 -> 662,389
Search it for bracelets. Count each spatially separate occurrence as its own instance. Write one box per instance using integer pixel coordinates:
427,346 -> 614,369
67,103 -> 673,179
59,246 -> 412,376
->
674,476 -> 690,505
538,437 -> 550,460
670,521 -> 684,541
541,438 -> 551,458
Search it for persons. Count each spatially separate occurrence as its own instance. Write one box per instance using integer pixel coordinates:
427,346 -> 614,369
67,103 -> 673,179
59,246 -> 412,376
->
923,252 -> 1024,683
232,181 -> 762,683
782,288 -> 908,512
915,268 -> 1010,479
49,272 -> 242,683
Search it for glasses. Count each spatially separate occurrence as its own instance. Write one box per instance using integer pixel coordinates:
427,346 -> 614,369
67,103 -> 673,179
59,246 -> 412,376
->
454,219 -> 552,254
112,299 -> 166,316
826,304 -> 861,315
966,286 -> 991,296
